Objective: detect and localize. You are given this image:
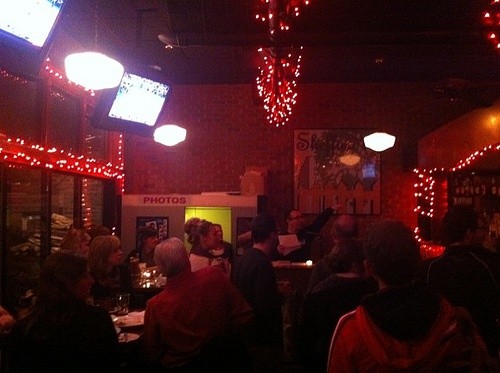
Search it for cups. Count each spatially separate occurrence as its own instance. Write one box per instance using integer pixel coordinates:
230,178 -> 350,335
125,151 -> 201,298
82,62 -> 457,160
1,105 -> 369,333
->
130,256 -> 139,277
116,293 -> 129,313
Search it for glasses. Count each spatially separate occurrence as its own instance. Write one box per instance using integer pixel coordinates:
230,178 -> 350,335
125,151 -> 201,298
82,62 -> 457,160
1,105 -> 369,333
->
289,215 -> 303,220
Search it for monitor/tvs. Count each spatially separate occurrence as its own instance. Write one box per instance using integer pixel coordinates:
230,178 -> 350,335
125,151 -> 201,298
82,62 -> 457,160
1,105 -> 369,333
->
0,0 -> 69,80
91,65 -> 174,137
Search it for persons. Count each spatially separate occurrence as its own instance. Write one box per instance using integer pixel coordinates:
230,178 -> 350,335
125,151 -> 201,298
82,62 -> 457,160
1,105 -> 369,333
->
0,205 -> 500,373
326,219 -> 500,373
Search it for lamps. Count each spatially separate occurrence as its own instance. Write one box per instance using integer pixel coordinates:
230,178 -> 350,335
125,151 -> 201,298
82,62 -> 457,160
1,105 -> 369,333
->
64,0 -> 125,90
